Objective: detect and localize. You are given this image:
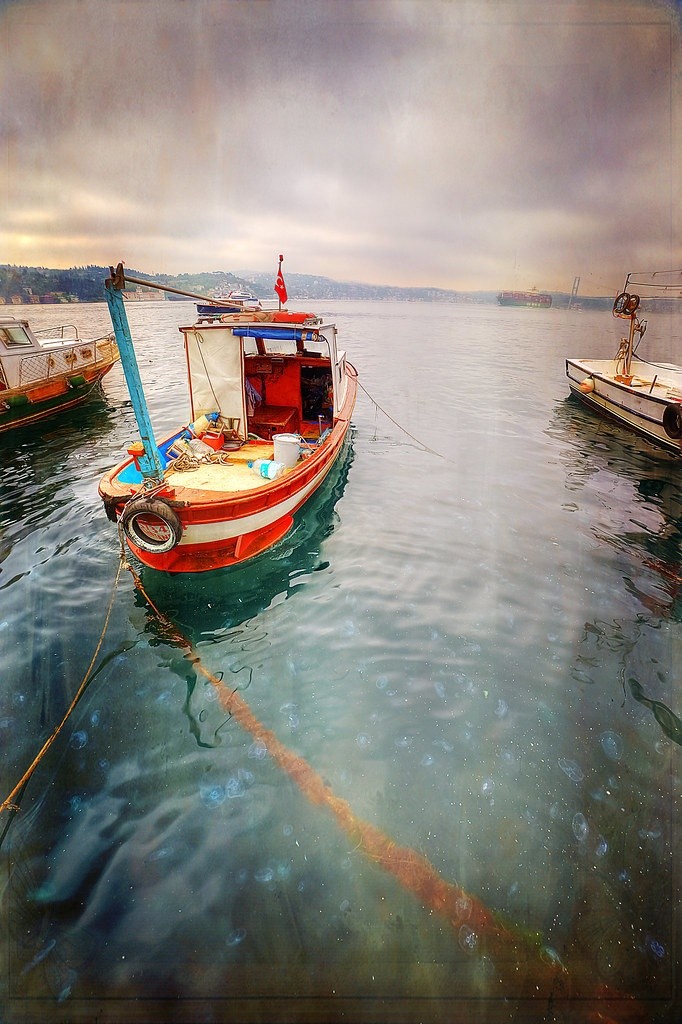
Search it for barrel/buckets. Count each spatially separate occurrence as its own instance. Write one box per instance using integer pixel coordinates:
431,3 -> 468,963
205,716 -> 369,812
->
272,432 -> 301,468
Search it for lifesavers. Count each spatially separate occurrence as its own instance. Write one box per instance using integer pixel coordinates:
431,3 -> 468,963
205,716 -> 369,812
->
122,499 -> 183,554
663,403 -> 682,439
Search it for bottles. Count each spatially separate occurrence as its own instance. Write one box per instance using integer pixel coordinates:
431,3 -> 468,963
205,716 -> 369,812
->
247,459 -> 287,481
185,439 -> 214,460
185,412 -> 219,437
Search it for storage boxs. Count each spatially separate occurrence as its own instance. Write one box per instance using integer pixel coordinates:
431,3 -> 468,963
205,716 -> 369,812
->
249,404 -> 299,439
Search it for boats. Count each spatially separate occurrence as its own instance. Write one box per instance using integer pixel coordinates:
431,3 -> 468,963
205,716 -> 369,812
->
565,293 -> 682,458
495,287 -> 556,309
103,251 -> 358,572
0,315 -> 122,434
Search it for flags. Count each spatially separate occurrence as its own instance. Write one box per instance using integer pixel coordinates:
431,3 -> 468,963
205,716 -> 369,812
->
274,269 -> 288,304
